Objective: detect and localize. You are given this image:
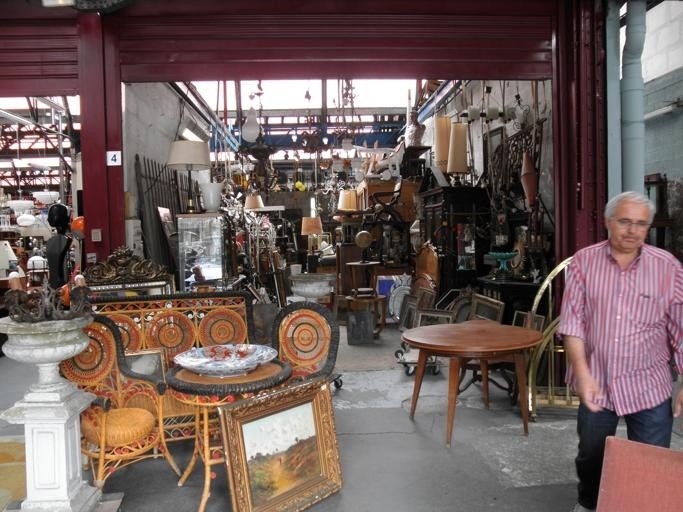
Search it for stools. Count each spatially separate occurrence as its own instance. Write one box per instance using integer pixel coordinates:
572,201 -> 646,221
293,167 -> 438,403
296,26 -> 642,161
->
456,319 -> 513,400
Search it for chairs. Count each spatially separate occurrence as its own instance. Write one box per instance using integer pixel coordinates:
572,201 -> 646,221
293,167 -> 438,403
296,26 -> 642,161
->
271,301 -> 340,386
60,314 -> 183,492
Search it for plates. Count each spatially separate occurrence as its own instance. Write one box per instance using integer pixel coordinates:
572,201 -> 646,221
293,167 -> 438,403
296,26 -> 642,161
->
174,344 -> 278,378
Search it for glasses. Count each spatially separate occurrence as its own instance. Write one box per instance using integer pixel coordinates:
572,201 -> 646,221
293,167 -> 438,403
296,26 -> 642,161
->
614,218 -> 651,229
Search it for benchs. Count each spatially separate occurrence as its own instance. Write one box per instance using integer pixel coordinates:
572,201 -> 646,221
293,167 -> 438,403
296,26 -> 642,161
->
87,289 -> 260,460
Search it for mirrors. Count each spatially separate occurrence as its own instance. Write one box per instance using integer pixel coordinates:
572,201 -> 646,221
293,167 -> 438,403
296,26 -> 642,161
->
177,217 -> 224,292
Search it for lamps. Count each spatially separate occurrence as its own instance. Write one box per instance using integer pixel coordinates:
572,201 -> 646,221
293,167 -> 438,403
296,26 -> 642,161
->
0,122 -> 70,280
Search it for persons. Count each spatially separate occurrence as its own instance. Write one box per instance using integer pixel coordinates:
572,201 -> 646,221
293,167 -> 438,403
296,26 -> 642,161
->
555,190 -> 683,512
44,204 -> 69,288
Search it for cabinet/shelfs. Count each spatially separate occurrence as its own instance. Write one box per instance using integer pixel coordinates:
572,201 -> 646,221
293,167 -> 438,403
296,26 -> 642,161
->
419,186 -> 491,310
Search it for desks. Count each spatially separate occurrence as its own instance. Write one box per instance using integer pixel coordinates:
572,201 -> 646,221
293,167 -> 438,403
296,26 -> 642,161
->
400,324 -> 544,449
345,295 -> 387,339
165,357 -> 293,512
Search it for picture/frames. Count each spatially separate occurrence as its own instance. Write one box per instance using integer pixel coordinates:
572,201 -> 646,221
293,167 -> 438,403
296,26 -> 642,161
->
376,273 -> 546,334
70,277 -> 178,371
123,347 -> 171,389
217,374 -> 344,512
